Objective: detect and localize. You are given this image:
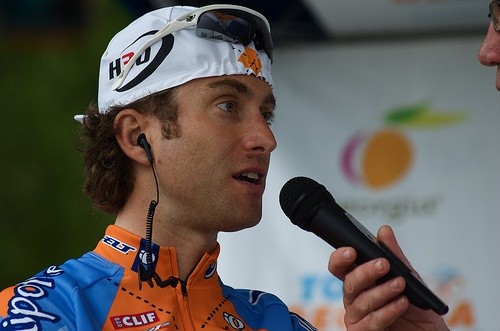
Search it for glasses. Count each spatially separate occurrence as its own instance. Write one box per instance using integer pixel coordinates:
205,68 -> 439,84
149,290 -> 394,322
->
488,0 -> 500,32
111,4 -> 273,92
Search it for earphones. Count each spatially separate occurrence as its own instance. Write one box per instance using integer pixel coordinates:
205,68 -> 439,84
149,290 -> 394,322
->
137,133 -> 151,151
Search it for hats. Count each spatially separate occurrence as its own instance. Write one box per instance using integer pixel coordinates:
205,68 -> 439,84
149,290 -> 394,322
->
74,6 -> 273,124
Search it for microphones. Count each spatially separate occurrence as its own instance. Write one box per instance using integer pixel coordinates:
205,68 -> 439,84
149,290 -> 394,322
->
279,176 -> 449,315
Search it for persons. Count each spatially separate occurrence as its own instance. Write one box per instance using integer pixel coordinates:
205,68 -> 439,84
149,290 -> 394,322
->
0,4 -> 321,331
327,0 -> 500,331
